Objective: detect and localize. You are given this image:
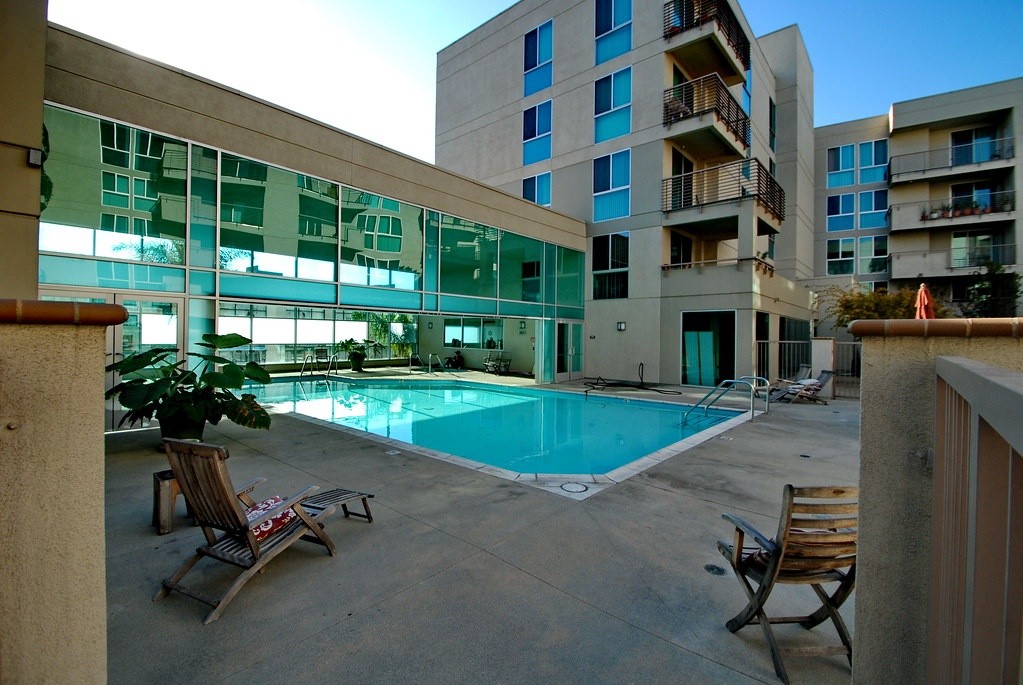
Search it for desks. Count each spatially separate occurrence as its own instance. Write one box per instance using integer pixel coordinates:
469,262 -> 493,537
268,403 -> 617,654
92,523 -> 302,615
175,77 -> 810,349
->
152,471 -> 199,536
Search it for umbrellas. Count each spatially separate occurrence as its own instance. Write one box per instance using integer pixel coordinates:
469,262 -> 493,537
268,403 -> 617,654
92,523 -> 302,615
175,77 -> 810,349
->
914,282 -> 934,318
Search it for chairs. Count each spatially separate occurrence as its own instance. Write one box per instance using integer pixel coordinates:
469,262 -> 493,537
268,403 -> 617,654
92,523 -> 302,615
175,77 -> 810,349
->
151,437 -> 374,625
716,483 -> 859,685
764,365 -> 813,403
778,369 -> 835,404
483,350 -> 513,376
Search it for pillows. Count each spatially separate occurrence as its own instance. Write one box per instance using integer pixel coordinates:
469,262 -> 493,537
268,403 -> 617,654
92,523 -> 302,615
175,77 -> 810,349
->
798,379 -> 821,386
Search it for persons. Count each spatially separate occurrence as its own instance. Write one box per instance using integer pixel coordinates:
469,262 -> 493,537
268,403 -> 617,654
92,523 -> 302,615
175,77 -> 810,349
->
444,350 -> 465,370
487,337 -> 496,349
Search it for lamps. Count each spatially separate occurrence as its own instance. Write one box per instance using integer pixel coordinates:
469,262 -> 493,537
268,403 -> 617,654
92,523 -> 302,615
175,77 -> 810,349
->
519,321 -> 526,329
428,323 -> 433,329
617,321 -> 625,331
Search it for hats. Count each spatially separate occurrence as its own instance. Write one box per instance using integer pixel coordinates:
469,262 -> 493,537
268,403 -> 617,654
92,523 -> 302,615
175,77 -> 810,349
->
454,351 -> 460,353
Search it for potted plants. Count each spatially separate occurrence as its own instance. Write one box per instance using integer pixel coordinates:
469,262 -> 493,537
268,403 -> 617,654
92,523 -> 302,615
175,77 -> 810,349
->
920,195 -> 1012,222
333,338 -> 386,373
103,329 -> 274,443
